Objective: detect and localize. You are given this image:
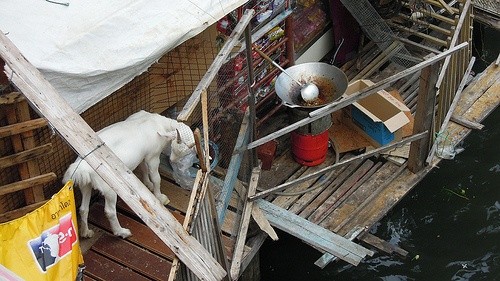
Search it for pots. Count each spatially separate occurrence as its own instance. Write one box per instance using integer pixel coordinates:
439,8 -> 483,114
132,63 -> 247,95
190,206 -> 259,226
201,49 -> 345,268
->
275,37 -> 348,108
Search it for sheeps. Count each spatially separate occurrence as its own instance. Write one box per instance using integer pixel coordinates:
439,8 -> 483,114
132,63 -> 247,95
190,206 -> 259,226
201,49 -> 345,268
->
60,110 -> 212,238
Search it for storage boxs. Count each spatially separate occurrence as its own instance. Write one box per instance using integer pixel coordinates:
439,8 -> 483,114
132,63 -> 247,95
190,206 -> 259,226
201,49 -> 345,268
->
341,79 -> 410,149
